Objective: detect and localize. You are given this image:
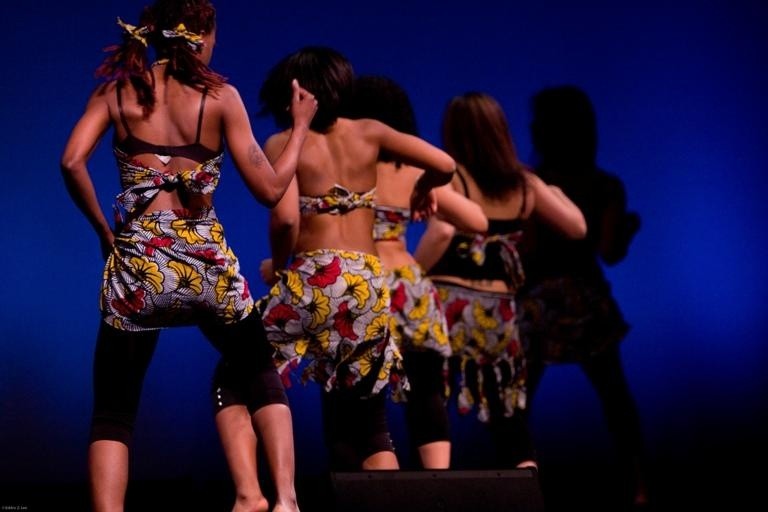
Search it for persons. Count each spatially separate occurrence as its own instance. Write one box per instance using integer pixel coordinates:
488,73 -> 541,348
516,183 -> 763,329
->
414,94 -> 590,469
212,44 -> 456,511
341,68 -> 488,471
517,87 -> 657,505
58,3 -> 318,512
